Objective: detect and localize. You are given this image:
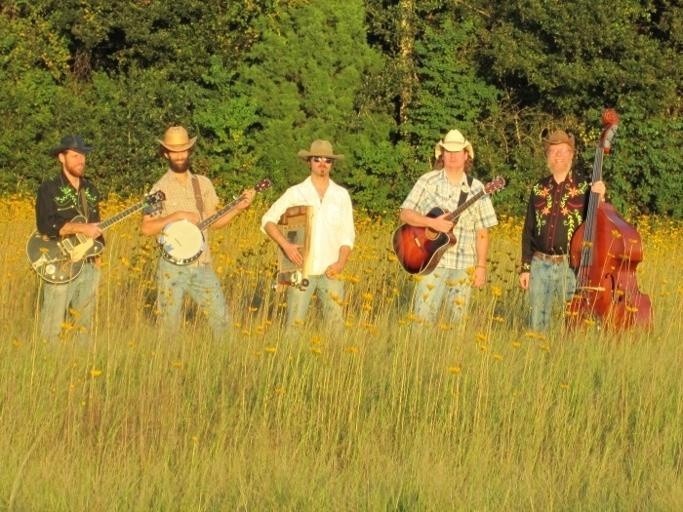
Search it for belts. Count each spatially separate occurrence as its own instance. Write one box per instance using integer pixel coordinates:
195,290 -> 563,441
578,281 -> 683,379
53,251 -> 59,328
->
533,251 -> 569,264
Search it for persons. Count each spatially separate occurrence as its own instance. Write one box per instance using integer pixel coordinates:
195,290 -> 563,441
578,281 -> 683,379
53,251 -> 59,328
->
35,135 -> 104,338
400,128 -> 497,335
141,126 -> 257,330
520,127 -> 605,336
262,139 -> 354,337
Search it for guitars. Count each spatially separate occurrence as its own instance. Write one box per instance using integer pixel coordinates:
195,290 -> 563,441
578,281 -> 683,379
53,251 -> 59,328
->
26,190 -> 166,284
392,175 -> 511,276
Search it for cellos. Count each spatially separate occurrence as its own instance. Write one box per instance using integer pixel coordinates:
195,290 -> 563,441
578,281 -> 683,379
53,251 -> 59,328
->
565,108 -> 654,344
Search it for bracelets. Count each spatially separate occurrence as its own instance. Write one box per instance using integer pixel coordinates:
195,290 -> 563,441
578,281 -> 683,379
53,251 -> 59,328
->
476,264 -> 487,269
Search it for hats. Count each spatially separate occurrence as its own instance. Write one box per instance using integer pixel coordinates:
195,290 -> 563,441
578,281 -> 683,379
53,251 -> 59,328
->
157,126 -> 198,152
48,136 -> 95,157
545,130 -> 568,149
298,140 -> 344,161
435,129 -> 475,161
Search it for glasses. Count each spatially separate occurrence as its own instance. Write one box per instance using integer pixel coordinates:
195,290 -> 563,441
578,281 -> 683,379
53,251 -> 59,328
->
311,156 -> 333,164
546,147 -> 572,156
